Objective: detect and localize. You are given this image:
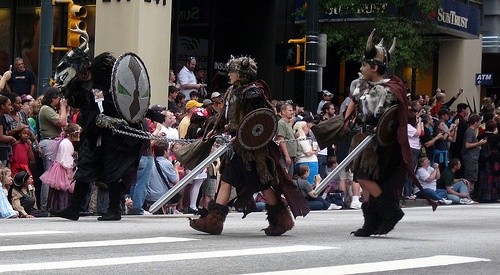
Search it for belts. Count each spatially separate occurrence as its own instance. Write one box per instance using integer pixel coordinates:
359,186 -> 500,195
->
41,137 -> 56,140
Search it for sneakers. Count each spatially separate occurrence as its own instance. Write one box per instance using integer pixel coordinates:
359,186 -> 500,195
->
460,198 -> 474,204
439,198 -> 453,205
349,196 -> 362,210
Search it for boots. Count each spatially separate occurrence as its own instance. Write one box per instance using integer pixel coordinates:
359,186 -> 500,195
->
353,202 -> 380,237
190,202 -> 229,235
373,191 -> 405,235
97,185 -> 121,220
49,181 -> 89,221
263,201 -> 294,236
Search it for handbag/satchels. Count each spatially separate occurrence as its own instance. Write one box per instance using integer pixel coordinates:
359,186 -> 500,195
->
170,193 -> 182,205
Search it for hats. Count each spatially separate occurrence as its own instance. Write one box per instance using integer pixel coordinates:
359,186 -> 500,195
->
301,111 -> 325,123
321,90 -> 334,99
364,29 -> 396,67
438,108 -> 453,117
151,104 -> 167,111
458,103 -> 469,113
185,91 -> 222,110
228,55 -> 257,75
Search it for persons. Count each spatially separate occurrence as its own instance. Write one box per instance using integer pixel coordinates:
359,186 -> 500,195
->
186,55 -> 294,236
271,90 -> 364,210
39,123 -> 82,211
402,87 -> 500,205
55,47 -> 151,222
12,57 -> 35,96
347,44 -> 405,236
177,57 -> 201,100
0,94 -> 82,185
143,68 -> 225,214
0,70 -> 12,93
0,167 -> 38,218
39,88 -> 68,217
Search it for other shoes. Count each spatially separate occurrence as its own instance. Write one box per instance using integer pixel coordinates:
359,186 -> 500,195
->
329,203 -> 342,210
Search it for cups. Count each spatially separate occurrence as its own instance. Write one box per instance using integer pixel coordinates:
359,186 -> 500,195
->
313,142 -> 318,150
